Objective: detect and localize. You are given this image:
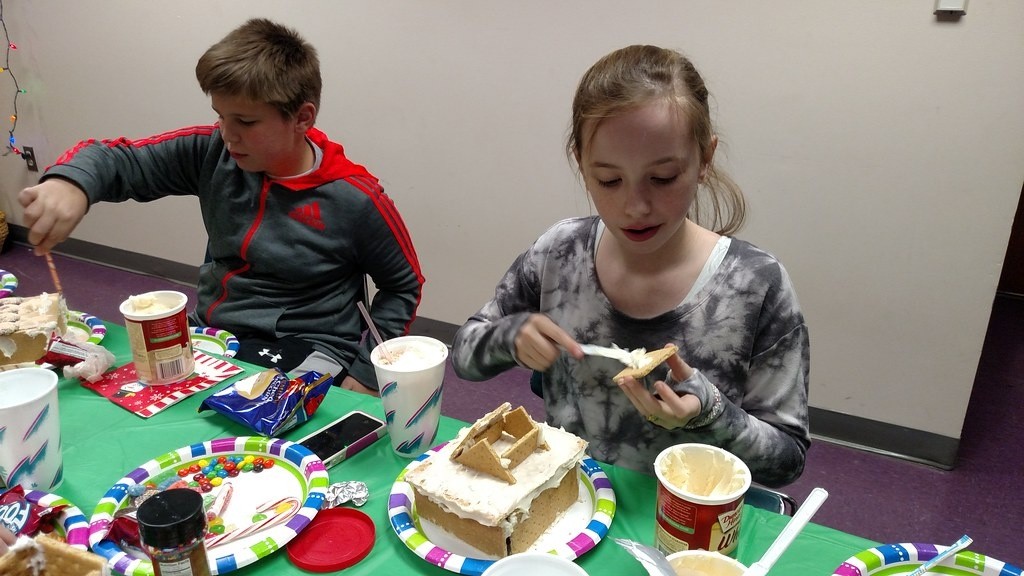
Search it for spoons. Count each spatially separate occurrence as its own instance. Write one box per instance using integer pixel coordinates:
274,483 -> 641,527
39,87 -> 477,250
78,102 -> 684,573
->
742,487 -> 829,576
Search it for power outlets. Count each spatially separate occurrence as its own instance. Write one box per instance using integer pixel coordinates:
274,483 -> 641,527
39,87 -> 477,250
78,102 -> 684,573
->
22,144 -> 40,174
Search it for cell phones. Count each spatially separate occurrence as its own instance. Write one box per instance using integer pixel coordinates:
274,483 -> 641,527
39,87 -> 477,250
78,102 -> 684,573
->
294,408 -> 387,470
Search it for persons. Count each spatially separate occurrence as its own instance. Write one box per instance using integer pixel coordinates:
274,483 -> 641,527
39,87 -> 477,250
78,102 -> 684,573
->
17,17 -> 425,398
450,45 -> 810,490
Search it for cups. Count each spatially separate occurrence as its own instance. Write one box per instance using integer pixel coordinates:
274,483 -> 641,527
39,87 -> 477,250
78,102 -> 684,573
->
370,335 -> 449,458
119,291 -> 195,386
650,441 -> 751,561
0,367 -> 65,494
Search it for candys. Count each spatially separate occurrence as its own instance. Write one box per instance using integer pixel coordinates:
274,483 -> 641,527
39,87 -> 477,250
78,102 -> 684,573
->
127,457 -> 292,538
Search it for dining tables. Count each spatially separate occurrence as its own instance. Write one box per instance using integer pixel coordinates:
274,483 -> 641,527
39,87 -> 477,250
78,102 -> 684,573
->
0,308 -> 884,576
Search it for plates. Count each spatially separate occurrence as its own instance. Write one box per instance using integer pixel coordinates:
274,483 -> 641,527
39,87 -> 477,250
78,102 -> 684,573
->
0,486 -> 88,552
88,435 -> 329,576
187,325 -> 240,359
65,308 -> 106,352
829,541 -> 1024,576
386,437 -> 616,575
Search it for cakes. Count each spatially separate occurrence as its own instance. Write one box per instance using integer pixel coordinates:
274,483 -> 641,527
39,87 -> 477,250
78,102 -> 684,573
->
0,290 -> 62,365
405,401 -> 590,559
